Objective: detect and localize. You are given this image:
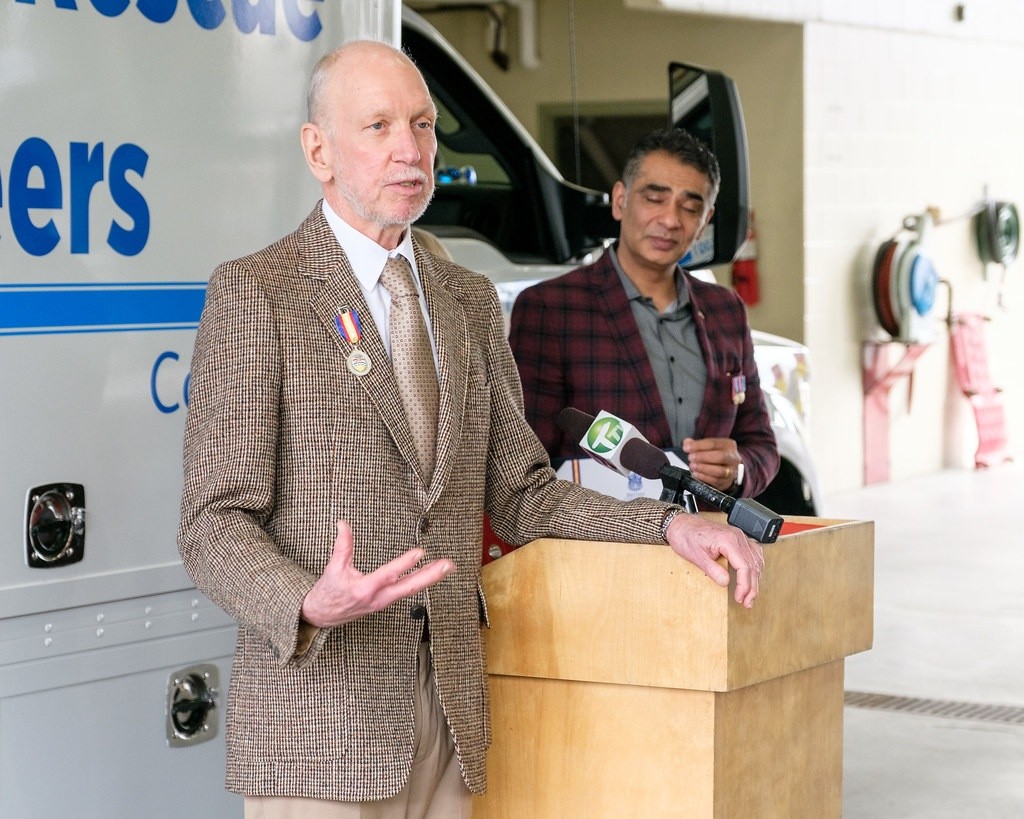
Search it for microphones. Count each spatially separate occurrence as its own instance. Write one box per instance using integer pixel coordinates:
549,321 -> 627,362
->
556,408 -> 784,543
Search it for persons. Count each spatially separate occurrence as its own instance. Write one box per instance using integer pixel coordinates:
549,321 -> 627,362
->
176,38 -> 769,819
505,125 -> 779,513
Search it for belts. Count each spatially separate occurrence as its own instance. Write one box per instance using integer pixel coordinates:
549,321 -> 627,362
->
421,623 -> 429,642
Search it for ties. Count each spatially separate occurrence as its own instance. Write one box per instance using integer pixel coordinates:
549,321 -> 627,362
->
378,254 -> 440,488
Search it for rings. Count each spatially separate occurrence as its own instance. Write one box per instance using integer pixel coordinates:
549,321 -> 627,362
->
725,466 -> 730,478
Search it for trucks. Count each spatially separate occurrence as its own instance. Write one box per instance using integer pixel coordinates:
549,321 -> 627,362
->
0,2 -> 816,819
